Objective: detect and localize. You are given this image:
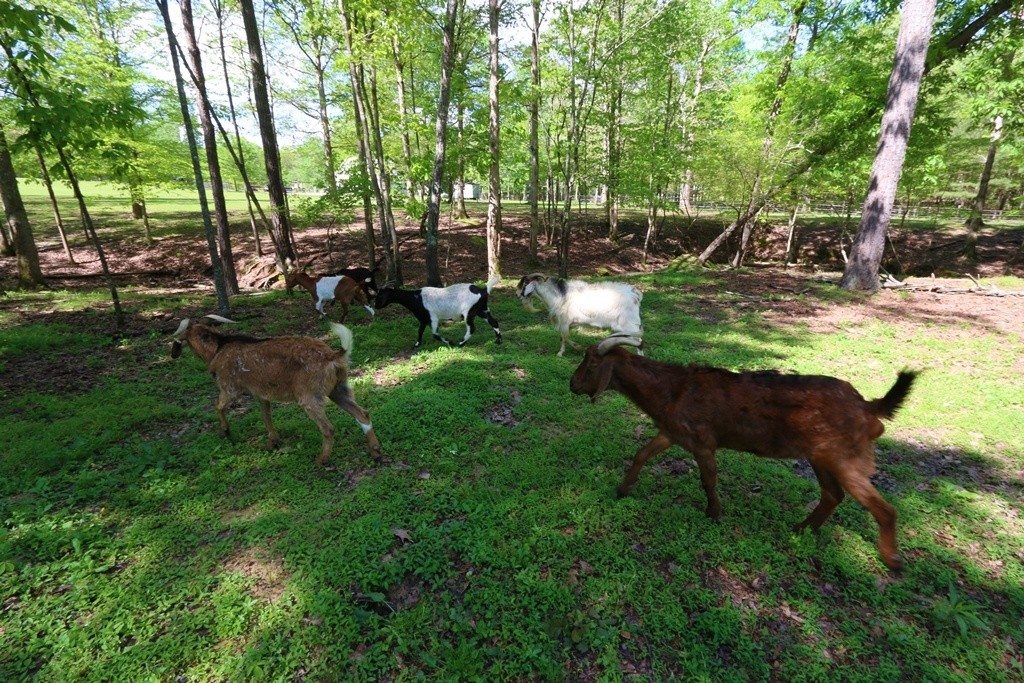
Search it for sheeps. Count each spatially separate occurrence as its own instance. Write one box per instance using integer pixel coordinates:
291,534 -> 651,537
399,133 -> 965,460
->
164,314 -> 392,466
375,284 -> 503,348
515,274 -> 647,358
287,264 -> 377,326
569,332 -> 924,573
